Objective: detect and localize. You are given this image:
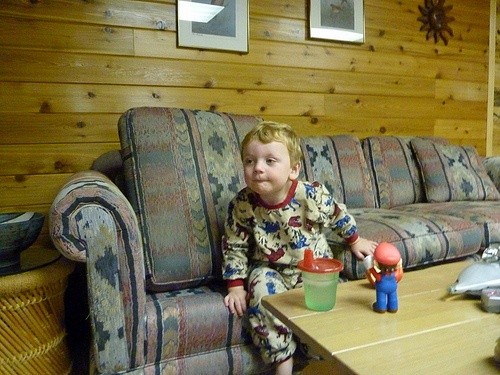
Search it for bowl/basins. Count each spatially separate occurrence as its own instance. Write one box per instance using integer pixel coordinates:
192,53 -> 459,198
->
0,212 -> 46,267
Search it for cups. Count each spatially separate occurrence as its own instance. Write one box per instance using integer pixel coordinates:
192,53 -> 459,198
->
297,249 -> 343,311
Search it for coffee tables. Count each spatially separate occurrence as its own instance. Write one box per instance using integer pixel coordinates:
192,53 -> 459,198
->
261,245 -> 499,375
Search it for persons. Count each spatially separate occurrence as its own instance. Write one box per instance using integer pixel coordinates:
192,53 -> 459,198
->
365,241 -> 404,314
220,122 -> 379,375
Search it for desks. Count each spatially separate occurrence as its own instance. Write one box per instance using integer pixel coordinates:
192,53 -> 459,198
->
0,239 -> 75,375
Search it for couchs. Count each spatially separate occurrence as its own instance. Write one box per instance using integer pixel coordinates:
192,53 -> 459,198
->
46,106 -> 499,375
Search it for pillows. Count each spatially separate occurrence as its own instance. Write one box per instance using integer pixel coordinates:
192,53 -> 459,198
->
359,133 -> 428,210
115,106 -> 280,294
295,130 -> 379,210
410,136 -> 500,204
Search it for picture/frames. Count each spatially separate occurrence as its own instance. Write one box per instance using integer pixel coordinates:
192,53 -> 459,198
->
174,0 -> 251,55
304,0 -> 367,46
